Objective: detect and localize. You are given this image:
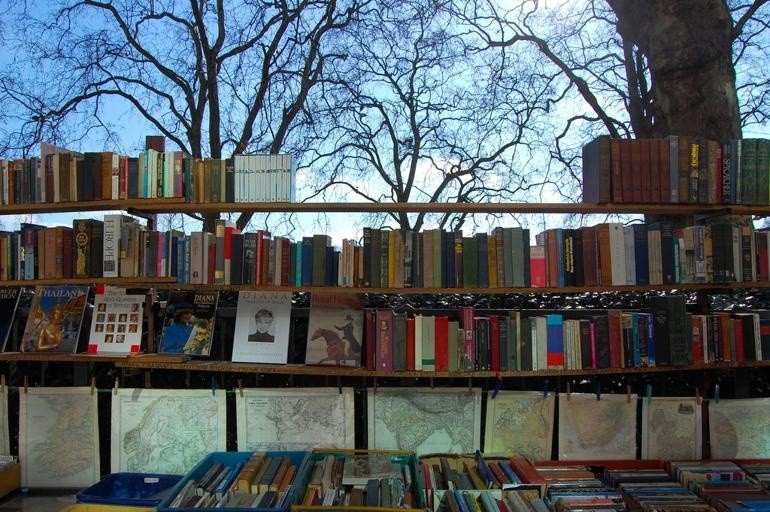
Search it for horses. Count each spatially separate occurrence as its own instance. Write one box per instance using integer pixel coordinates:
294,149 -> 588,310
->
310,328 -> 361,367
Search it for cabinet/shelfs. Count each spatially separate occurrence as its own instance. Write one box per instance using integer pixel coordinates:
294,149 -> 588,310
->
1,199 -> 770,379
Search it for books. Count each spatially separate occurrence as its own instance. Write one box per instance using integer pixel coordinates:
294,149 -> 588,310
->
585,135 -> 770,205
0,135 -> 296,202
80,447 -> 770,512
0,213 -> 770,371
